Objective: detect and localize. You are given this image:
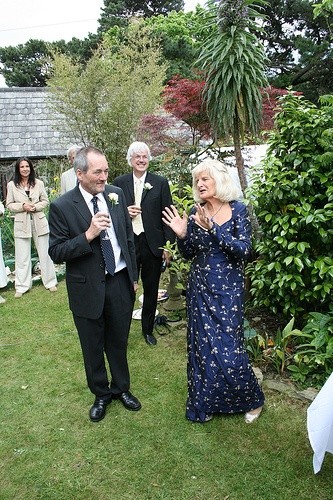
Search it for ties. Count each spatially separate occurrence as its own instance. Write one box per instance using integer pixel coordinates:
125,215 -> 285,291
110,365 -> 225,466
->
132,180 -> 142,236
91,197 -> 116,277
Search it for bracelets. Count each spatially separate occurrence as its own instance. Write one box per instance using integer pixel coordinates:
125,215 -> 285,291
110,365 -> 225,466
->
23,203 -> 25,206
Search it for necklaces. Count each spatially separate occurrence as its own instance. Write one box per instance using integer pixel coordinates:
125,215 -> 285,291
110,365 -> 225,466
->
207,199 -> 225,221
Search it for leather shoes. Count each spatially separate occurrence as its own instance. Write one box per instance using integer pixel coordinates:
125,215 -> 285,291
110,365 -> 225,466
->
89,396 -> 112,422
112,391 -> 142,411
142,331 -> 157,346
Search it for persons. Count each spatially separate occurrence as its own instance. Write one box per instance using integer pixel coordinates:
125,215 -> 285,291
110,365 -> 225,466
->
111,142 -> 173,346
48,147 -> 143,423
0,146 -> 83,308
161,160 -> 264,424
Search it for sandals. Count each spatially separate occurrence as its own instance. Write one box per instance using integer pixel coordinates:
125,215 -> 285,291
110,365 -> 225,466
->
204,414 -> 214,421
245,404 -> 262,424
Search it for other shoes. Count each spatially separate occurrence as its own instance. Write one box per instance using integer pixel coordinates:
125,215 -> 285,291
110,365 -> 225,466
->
49,286 -> 57,292
15,292 -> 22,298
0,297 -> 6,304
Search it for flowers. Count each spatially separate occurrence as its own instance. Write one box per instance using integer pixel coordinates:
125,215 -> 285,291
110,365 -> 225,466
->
107,191 -> 119,208
142,181 -> 153,192
